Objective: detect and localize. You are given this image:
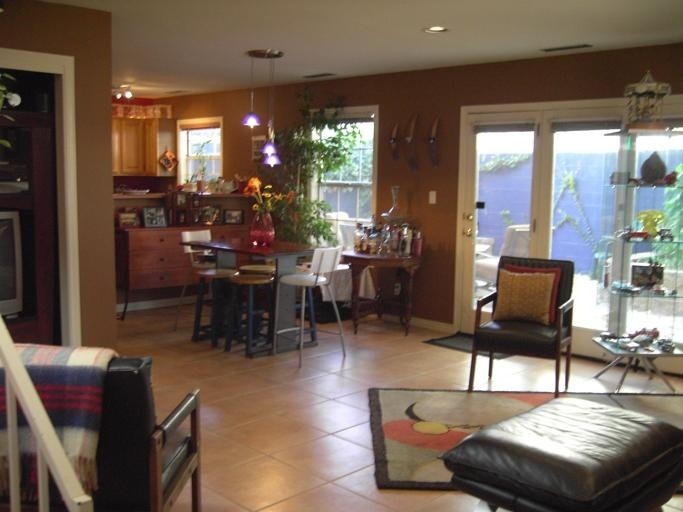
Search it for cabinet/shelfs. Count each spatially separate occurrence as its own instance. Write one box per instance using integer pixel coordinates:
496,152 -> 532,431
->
0,107 -> 61,352
115,221 -> 255,293
111,117 -> 140,176
602,131 -> 683,361
141,117 -> 176,179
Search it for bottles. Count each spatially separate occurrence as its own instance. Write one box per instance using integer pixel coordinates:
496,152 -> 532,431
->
352,221 -> 423,257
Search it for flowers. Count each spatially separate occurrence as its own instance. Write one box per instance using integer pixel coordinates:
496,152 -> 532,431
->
242,176 -> 278,230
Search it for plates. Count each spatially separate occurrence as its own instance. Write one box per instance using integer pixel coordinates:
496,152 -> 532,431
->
119,188 -> 150,195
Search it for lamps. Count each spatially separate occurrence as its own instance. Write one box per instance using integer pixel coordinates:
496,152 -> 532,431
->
115,83 -> 133,98
239,47 -> 284,169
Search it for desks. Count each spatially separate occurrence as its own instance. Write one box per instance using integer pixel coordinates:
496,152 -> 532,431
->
180,237 -> 324,354
339,218 -> 372,247
592,334 -> 682,394
304,262 -> 352,324
343,250 -> 421,337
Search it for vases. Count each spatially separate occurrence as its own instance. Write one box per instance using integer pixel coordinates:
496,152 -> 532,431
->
247,211 -> 274,246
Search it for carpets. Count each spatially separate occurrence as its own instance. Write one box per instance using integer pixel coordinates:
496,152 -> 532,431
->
365,382 -> 682,490
420,327 -> 514,362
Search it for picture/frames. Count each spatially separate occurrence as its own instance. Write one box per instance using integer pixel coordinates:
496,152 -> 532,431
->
140,206 -> 167,228
118,211 -> 139,229
222,208 -> 244,224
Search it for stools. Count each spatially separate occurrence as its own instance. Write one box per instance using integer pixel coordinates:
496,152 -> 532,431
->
220,272 -> 275,361
192,269 -> 238,349
295,265 -> 315,342
239,262 -> 275,344
434,395 -> 683,512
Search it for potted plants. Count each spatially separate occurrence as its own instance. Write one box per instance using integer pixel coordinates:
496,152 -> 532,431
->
183,179 -> 193,193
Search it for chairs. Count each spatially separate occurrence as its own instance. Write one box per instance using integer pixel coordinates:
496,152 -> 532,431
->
0,341 -> 201,511
324,211 -> 349,243
473,231 -> 494,255
272,244 -> 347,368
473,224 -> 558,290
169,229 -> 217,332
468,255 -> 576,398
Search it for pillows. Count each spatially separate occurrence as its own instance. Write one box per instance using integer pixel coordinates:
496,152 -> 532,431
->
502,264 -> 565,324
494,270 -> 554,327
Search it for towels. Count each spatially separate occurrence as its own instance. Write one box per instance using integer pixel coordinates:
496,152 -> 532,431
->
359,264 -> 383,300
